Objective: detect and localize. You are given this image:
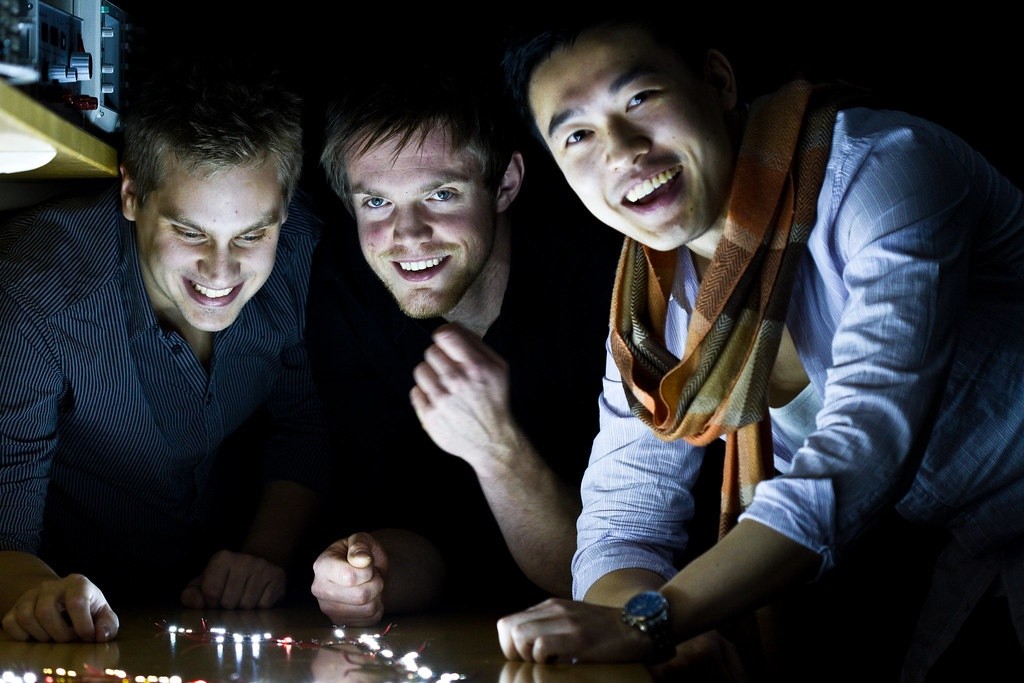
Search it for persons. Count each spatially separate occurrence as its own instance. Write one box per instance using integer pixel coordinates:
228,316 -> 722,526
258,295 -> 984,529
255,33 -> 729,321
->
495,9 -> 1024,683
0,87 -> 620,683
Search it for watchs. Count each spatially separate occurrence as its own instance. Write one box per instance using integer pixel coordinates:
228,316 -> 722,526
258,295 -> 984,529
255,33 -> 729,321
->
621,588 -> 677,664
